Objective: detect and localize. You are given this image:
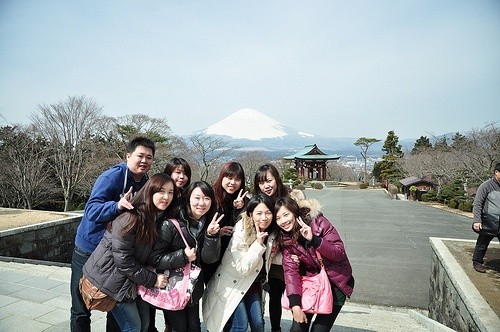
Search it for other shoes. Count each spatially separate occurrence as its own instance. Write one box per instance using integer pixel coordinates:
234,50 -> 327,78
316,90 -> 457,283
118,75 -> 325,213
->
473,262 -> 486,273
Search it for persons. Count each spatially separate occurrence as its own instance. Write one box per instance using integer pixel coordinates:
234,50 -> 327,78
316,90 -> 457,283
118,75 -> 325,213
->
202,194 -> 280,332
70,137 -> 155,332
162,158 -> 191,198
273,197 -> 355,332
149,180 -> 224,332
253,164 -> 305,332
78,173 -> 178,332
202,162 -> 250,286
471,163 -> 500,272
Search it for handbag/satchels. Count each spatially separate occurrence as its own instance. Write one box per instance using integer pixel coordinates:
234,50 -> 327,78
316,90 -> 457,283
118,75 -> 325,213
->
281,266 -> 334,314
77,275 -> 117,313
134,260 -> 201,311
472,211 -> 500,238
